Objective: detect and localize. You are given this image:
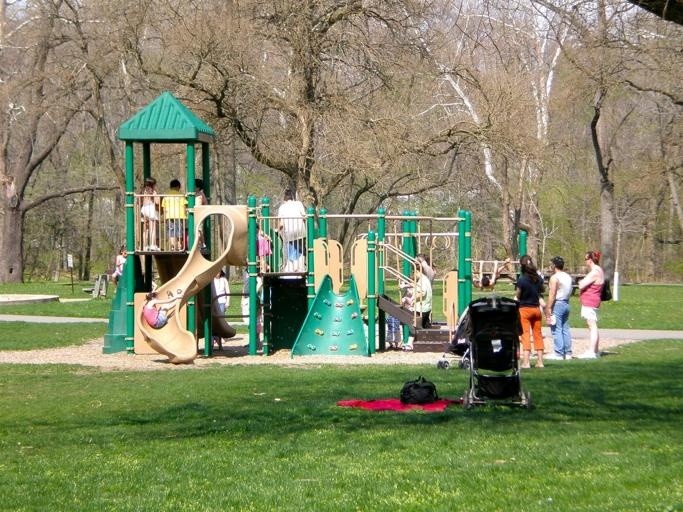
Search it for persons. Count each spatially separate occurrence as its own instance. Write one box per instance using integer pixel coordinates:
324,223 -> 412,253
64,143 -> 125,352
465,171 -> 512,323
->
471,257 -> 511,288
400,287 -> 413,347
576,249 -> 605,359
545,257 -> 574,360
410,253 -> 435,284
512,296 -> 546,356
411,269 -> 432,329
110,178 -> 311,350
512,255 -> 545,367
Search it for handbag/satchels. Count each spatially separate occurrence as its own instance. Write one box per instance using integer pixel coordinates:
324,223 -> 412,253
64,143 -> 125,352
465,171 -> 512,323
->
400,376 -> 438,405
601,280 -> 611,302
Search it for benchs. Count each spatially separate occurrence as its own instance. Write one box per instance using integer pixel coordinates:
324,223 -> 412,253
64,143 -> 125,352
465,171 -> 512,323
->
474,270 -> 587,297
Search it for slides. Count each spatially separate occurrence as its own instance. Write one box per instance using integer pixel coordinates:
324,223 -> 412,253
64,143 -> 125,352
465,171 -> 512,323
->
291,276 -> 368,355
137,205 -> 249,363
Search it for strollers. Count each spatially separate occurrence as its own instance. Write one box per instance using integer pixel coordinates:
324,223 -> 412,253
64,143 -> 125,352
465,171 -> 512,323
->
462,296 -> 531,414
436,304 -> 479,370
